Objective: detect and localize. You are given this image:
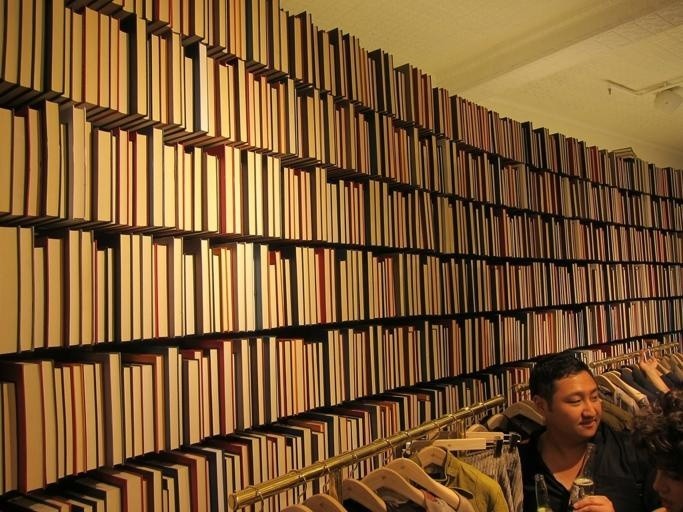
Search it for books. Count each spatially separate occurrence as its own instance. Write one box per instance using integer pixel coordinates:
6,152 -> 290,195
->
2,0 -> 682,512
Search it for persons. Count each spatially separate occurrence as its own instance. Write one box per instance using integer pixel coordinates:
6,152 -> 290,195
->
514,353 -> 661,512
630,389 -> 683,512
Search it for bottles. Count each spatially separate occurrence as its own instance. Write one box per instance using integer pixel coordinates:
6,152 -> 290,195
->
534,473 -> 554,512
567,442 -> 598,512
